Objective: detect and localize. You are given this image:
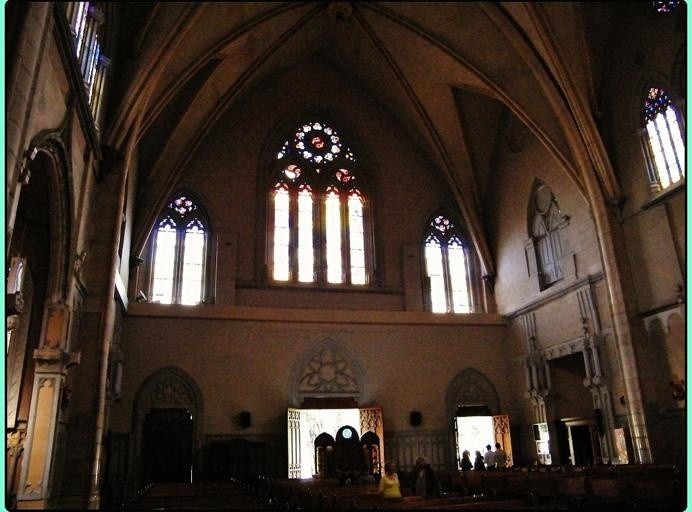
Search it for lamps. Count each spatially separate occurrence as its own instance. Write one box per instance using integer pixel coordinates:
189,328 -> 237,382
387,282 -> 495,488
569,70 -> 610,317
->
525,385 -> 539,400
583,374 -> 603,388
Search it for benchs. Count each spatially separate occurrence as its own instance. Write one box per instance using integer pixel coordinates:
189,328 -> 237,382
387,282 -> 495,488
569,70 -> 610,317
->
112,456 -> 686,511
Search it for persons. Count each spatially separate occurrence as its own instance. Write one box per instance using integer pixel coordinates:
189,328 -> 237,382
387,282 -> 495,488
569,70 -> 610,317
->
494,442 -> 507,469
459,449 -> 474,470
379,461 -> 401,498
412,456 -> 442,499
472,450 -> 487,471
483,444 -> 497,470
